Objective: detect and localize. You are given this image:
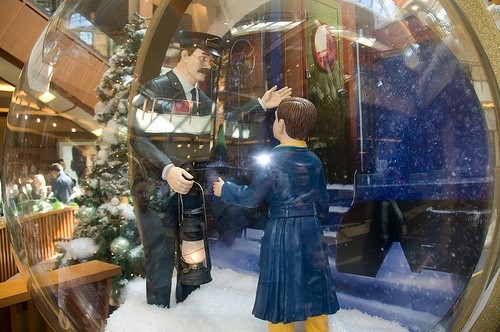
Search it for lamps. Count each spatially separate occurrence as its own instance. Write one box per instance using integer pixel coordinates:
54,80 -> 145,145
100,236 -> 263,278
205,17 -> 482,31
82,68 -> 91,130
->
179,181 -> 213,287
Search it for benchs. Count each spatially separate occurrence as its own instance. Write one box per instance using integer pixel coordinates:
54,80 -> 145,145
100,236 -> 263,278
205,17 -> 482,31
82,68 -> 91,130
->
0,260 -> 122,332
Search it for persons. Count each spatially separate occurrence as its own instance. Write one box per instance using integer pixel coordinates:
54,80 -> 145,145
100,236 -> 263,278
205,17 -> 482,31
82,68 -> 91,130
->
0,158 -> 97,207
213,96 -> 339,331
130,29 -> 292,311
